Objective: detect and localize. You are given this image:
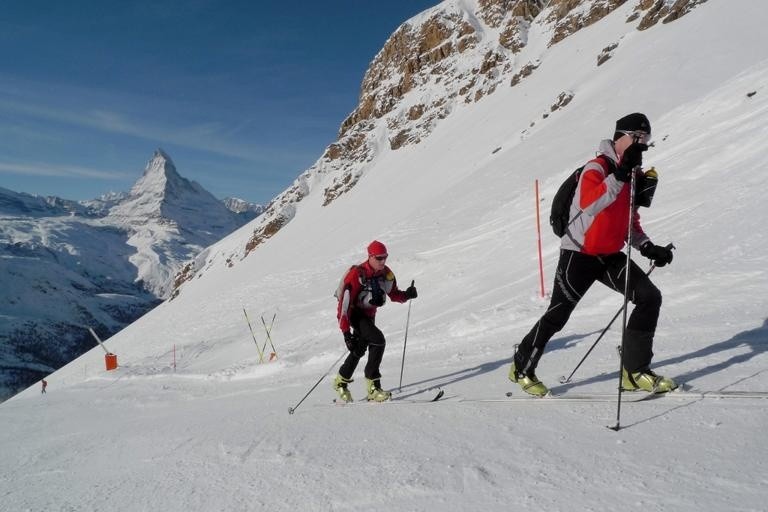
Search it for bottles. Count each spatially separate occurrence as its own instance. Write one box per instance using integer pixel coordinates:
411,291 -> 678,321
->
636,166 -> 657,205
385,271 -> 394,293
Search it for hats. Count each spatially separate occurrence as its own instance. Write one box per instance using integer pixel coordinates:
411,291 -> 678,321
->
367,240 -> 387,256
615,112 -> 651,135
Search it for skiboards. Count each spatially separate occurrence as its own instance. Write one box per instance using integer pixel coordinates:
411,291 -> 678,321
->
458,385 -> 768,399
314,391 -> 444,406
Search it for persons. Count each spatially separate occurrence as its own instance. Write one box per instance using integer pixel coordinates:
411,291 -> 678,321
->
333,239 -> 419,401
40,377 -> 47,393
507,112 -> 677,398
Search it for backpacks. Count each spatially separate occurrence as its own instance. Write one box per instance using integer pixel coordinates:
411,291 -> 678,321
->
549,156 -> 615,239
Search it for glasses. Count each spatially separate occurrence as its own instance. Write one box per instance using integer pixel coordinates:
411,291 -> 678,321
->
374,254 -> 389,260
626,130 -> 652,145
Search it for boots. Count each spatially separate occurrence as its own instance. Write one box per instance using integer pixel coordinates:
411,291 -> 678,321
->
332,370 -> 354,403
367,378 -> 392,401
508,356 -> 548,396
616,346 -> 678,393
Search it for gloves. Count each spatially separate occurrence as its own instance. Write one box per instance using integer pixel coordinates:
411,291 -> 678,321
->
640,240 -> 673,267
615,143 -> 649,181
406,287 -> 418,299
344,330 -> 359,350
